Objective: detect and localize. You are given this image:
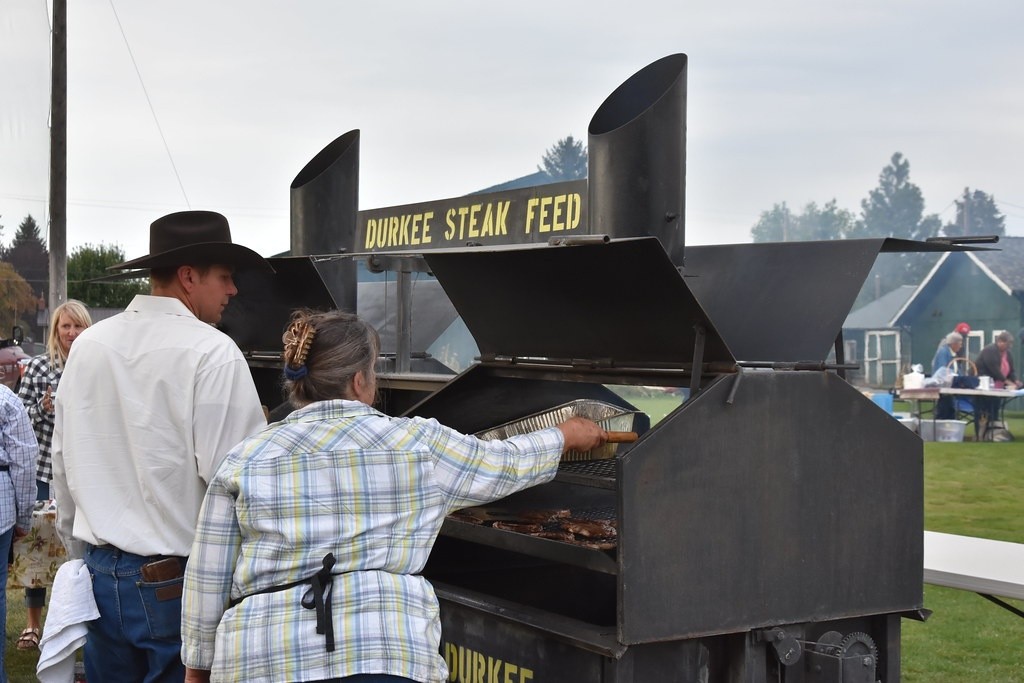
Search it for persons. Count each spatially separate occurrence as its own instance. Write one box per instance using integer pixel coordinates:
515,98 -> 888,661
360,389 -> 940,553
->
0,383 -> 41,683
180,313 -> 609,683
51,211 -> 268,683
932,323 -> 1022,435
17,300 -> 93,649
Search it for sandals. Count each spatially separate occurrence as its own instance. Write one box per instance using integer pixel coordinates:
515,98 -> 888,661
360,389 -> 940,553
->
16,627 -> 39,651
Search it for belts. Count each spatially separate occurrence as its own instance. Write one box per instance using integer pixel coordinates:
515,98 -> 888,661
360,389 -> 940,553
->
96,543 -> 126,552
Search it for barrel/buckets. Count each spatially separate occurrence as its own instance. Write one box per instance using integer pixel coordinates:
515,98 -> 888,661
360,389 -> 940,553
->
871,392 -> 893,415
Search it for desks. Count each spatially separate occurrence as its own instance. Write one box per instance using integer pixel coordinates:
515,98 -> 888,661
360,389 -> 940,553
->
923,530 -> 1024,619
899,387 -> 1020,442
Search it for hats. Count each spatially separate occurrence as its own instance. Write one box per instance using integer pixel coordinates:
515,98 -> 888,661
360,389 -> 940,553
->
105,211 -> 277,278
956,322 -> 970,335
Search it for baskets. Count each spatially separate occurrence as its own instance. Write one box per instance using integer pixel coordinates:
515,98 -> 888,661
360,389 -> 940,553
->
944,358 -> 980,389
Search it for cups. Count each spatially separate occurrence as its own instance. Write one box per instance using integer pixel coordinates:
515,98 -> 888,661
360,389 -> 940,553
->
979,376 -> 990,390
